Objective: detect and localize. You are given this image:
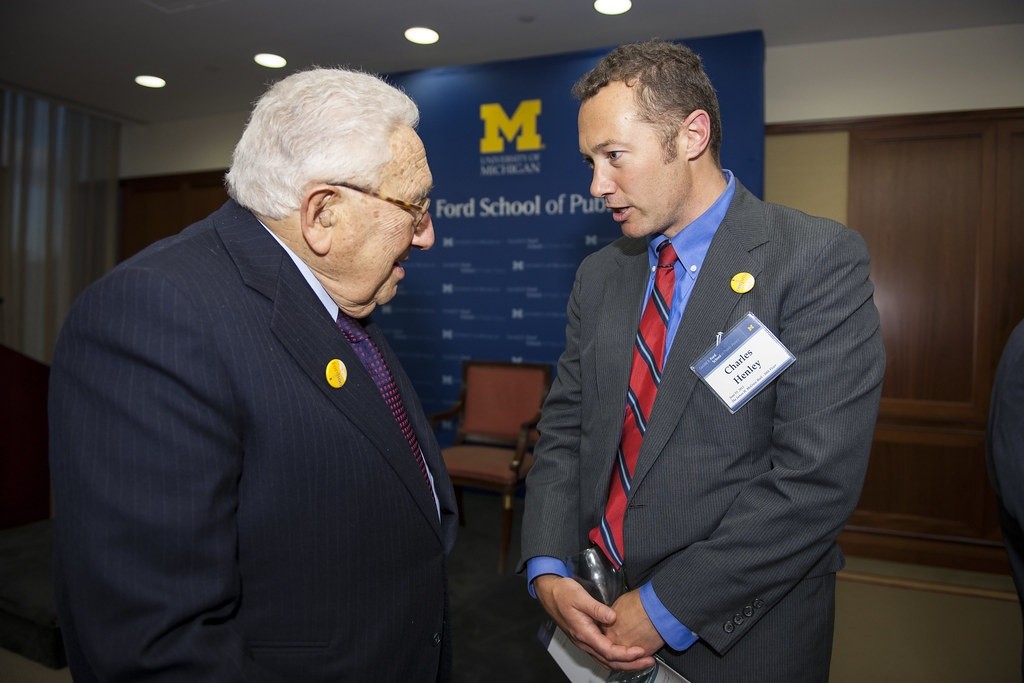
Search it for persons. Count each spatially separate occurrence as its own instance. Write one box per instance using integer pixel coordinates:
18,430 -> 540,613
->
48,68 -> 463,683
514,40 -> 885,683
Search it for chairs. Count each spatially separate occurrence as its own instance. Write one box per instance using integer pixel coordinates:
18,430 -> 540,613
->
430,360 -> 554,574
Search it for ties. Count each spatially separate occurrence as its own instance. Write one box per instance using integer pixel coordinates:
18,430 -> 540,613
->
334,314 -> 438,524
589,243 -> 678,572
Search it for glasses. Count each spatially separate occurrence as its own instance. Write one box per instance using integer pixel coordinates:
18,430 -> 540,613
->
328,181 -> 432,227
